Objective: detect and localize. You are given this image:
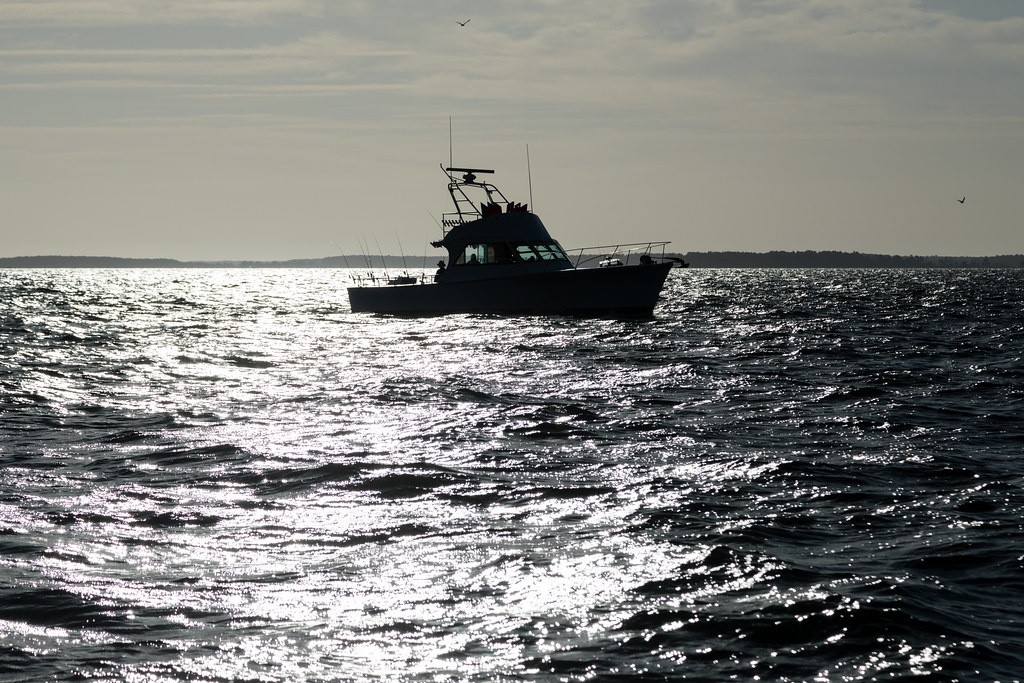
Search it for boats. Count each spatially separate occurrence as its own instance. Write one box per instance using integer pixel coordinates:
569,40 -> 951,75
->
343,115 -> 692,322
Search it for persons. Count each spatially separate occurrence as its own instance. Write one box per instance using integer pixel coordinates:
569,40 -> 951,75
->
434,260 -> 446,282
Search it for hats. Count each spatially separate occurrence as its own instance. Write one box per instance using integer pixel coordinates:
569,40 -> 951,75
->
437,261 -> 446,267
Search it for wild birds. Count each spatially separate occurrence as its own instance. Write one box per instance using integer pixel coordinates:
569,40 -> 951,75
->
956,196 -> 966,203
456,18 -> 471,26
